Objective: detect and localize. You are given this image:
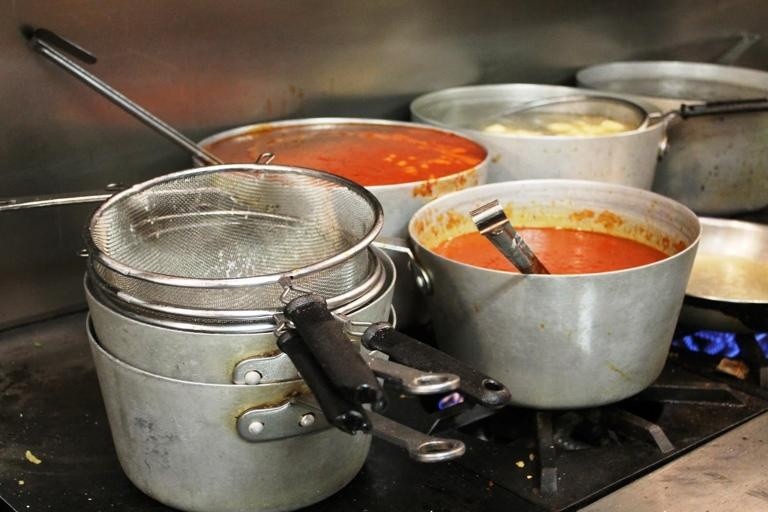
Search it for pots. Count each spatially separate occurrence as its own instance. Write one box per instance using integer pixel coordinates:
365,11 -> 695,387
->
579,60 -> 768,217
683,209 -> 768,360
79,222 -> 512,512
415,84 -> 668,203
407,177 -> 702,404
197,116 -> 488,294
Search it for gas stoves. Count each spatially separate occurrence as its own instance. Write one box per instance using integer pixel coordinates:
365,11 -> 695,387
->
374,298 -> 768,512
